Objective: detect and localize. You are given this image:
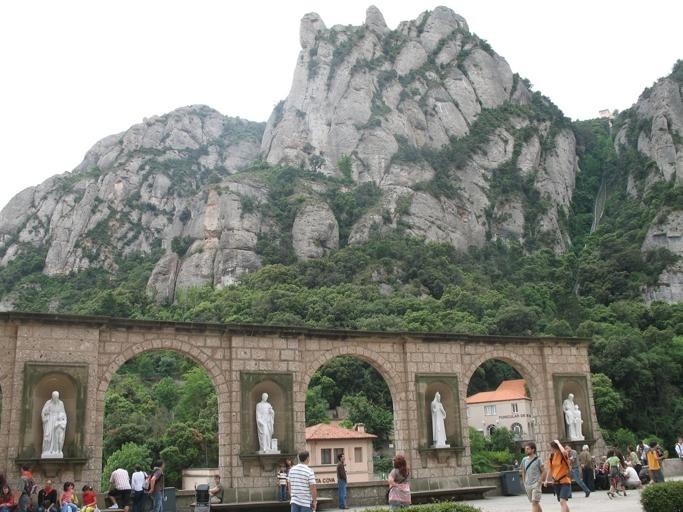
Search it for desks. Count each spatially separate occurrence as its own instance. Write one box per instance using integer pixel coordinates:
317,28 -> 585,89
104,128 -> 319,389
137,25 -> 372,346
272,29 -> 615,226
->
405,475 -> 497,502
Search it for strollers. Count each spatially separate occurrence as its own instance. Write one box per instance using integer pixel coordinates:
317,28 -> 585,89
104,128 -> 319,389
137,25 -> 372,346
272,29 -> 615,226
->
192,482 -> 211,512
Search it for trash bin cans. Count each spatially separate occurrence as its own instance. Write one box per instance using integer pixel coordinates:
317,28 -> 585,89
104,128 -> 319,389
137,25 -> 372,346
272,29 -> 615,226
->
161,487 -> 176,512
500,470 -> 521,496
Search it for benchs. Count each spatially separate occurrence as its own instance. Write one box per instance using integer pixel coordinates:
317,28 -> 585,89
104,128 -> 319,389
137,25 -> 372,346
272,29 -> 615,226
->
31,493 -> 126,512
192,496 -> 334,511
541,464 -> 648,493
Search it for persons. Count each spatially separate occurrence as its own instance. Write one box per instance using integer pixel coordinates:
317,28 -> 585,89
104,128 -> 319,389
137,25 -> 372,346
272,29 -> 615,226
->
277,463 -> 288,501
521,436 -> 683,512
387,455 -> 411,510
286,458 -> 296,500
0,460 -> 165,512
193,475 -> 223,505
562,393 -> 577,436
430,392 -> 447,445
575,404 -> 583,436
41,391 -> 67,453
54,413 -> 67,453
255,393 -> 275,451
337,453 -> 349,509
287,450 -> 317,512
